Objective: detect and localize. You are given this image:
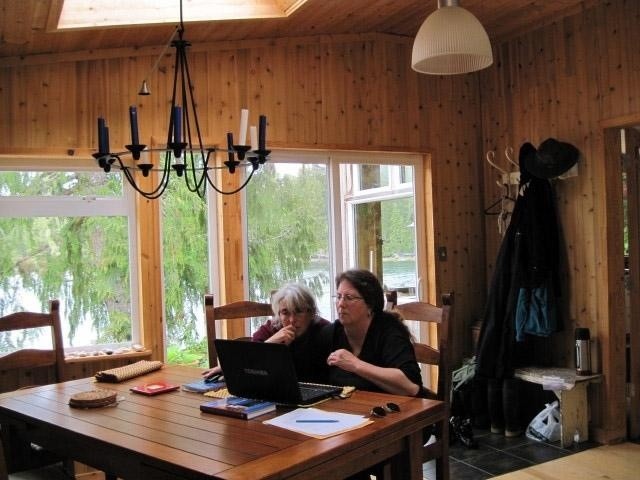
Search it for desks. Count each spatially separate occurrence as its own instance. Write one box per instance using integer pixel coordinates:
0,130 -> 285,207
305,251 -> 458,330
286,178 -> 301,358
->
515,368 -> 606,453
0,363 -> 448,479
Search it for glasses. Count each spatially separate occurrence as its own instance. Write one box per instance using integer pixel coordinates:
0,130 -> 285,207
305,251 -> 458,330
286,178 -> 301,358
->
370,402 -> 401,418
334,294 -> 364,299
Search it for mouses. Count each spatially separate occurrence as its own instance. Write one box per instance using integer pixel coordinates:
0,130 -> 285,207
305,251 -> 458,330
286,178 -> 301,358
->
206,374 -> 222,384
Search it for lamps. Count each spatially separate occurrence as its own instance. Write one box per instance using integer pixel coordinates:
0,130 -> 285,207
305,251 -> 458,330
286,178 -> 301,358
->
91,0 -> 270,200
409,1 -> 497,76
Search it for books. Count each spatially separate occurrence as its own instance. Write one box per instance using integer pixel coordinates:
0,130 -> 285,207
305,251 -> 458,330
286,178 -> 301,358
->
200,396 -> 276,419
131,382 -> 179,395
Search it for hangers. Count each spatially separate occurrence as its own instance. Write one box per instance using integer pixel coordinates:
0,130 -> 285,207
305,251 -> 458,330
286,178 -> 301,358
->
482,184 -> 518,216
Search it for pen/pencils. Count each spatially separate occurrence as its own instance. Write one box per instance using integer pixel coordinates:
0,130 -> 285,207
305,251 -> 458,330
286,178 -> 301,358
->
296,420 -> 340,423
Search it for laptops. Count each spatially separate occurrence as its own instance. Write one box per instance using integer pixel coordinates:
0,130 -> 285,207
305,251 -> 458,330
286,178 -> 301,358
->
214,339 -> 343,404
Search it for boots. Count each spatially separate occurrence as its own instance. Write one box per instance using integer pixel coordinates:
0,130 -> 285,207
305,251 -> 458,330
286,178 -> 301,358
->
485,377 -> 505,435
500,377 -> 525,438
446,413 -> 479,450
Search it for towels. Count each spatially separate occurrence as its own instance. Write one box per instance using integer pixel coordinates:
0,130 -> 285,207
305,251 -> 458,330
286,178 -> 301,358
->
95,358 -> 164,384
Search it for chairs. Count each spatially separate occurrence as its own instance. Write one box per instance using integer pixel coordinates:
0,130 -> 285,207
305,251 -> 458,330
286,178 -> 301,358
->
0,298 -> 79,479
372,289 -> 457,480
202,289 -> 279,369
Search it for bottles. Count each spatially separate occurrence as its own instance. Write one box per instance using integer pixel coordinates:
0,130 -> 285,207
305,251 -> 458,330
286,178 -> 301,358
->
575,326 -> 591,375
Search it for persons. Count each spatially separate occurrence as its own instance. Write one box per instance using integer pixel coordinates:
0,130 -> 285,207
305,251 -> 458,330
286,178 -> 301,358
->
201,270 -> 432,480
253,283 -> 331,345
